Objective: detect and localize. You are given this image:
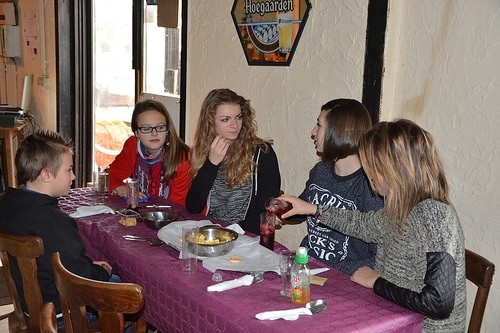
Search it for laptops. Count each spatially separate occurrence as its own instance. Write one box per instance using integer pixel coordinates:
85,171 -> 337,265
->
0,73 -> 33,115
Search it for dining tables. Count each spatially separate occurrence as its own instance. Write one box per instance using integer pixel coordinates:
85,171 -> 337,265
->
59,188 -> 426,333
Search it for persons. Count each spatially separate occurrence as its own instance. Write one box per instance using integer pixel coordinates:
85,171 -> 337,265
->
0,129 -> 132,333
286,99 -> 388,276
185,89 -> 281,234
104,99 -> 207,216
277,119 -> 467,333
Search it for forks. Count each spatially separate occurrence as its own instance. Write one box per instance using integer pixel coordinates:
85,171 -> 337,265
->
309,303 -> 326,314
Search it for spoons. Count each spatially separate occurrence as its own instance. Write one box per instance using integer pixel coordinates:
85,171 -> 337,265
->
252,271 -> 265,282
306,300 -> 327,314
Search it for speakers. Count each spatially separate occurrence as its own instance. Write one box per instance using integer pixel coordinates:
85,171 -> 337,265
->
0,25 -> 22,58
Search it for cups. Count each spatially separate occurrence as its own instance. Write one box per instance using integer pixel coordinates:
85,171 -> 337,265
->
182,225 -> 199,274
279,250 -> 296,297
92,172 -> 106,204
260,212 -> 276,251
99,165 -> 110,194
126,179 -> 140,210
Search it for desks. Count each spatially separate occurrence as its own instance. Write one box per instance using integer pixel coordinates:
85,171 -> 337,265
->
0,124 -> 28,191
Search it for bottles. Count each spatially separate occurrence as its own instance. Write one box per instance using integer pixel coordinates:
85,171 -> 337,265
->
290,247 -> 310,305
265,197 -> 308,225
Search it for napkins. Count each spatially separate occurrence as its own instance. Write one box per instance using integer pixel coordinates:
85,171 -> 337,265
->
69,205 -> 115,219
206,274 -> 254,293
299,268 -> 329,276
256,303 -> 312,322
224,223 -> 245,232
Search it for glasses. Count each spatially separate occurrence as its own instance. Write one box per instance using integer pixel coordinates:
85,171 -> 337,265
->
137,125 -> 169,133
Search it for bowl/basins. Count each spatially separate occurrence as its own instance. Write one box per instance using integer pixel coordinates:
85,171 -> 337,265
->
139,212 -> 177,231
185,225 -> 239,257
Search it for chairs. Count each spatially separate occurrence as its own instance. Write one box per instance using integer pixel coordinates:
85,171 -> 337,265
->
464,248 -> 495,333
0,233 -> 151,333
